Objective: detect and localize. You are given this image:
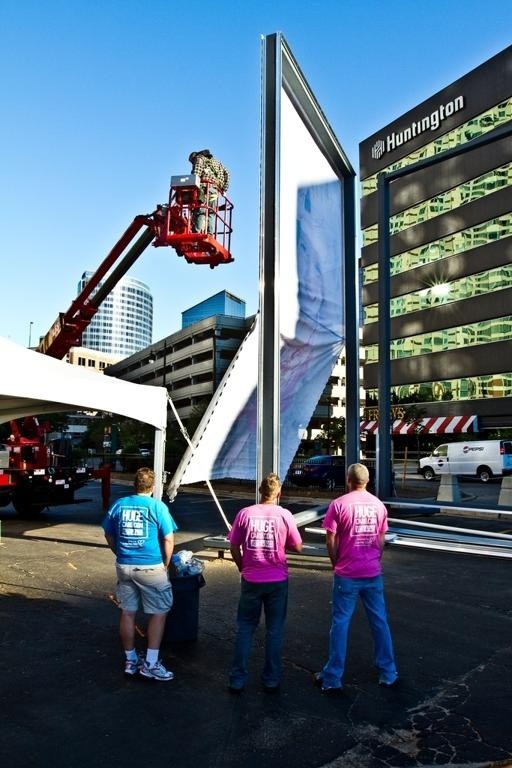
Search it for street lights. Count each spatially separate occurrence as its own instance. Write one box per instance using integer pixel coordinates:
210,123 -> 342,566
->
148,339 -> 167,385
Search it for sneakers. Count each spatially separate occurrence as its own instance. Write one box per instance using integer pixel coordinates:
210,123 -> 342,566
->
140,658 -> 174,681
124,657 -> 146,676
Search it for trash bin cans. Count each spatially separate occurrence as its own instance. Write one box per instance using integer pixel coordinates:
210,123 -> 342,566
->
165,559 -> 206,643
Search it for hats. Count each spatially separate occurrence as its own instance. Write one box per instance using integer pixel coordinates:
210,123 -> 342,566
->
189,149 -> 210,162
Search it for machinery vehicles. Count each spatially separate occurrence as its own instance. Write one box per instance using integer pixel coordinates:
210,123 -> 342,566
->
0,174 -> 235,521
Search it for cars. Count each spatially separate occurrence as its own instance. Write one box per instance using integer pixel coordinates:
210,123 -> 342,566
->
290,455 -> 376,491
139,448 -> 150,456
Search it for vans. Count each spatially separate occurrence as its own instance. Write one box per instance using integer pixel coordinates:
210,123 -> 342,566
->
417,438 -> 512,483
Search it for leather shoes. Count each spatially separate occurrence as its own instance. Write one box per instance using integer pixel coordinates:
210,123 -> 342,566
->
228,673 -> 408,698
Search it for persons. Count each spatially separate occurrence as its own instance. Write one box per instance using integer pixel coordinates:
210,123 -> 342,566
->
101,467 -> 179,682
312,463 -> 398,693
226,472 -> 303,695
